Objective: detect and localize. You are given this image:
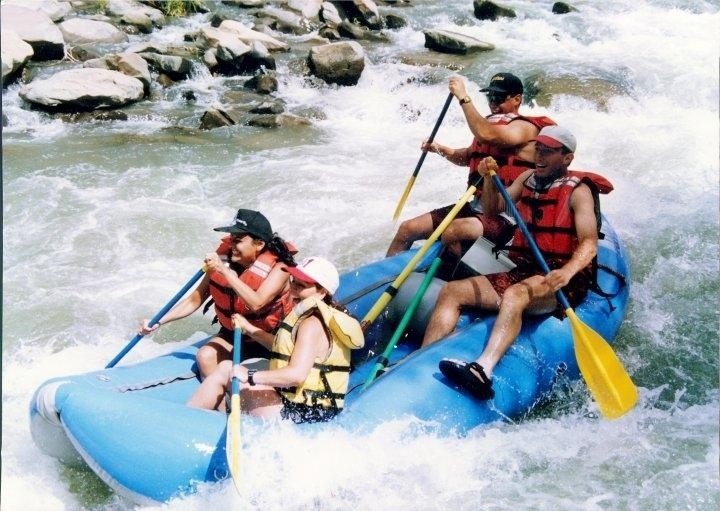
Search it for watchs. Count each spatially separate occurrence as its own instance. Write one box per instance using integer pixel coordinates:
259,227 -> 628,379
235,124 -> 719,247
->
459,94 -> 472,107
247,367 -> 259,386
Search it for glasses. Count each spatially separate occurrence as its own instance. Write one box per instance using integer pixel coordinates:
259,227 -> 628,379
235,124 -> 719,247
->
486,96 -> 511,104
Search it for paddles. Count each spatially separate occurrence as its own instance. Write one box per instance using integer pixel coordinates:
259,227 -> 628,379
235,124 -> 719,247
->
487,169 -> 639,421
225,321 -> 242,490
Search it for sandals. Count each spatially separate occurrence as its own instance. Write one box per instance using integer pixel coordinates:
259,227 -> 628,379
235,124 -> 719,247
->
439,358 -> 495,400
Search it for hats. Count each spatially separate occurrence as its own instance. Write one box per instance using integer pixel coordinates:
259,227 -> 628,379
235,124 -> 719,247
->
528,125 -> 577,152
478,73 -> 523,94
213,210 -> 272,240
281,256 -> 340,295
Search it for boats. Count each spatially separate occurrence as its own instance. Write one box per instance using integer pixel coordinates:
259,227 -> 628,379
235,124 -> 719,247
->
29,201 -> 632,506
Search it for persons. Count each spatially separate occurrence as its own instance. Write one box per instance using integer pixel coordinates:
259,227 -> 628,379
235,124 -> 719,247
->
137,207 -> 299,412
185,254 -> 369,425
386,72 -> 559,259
419,121 -> 616,401
